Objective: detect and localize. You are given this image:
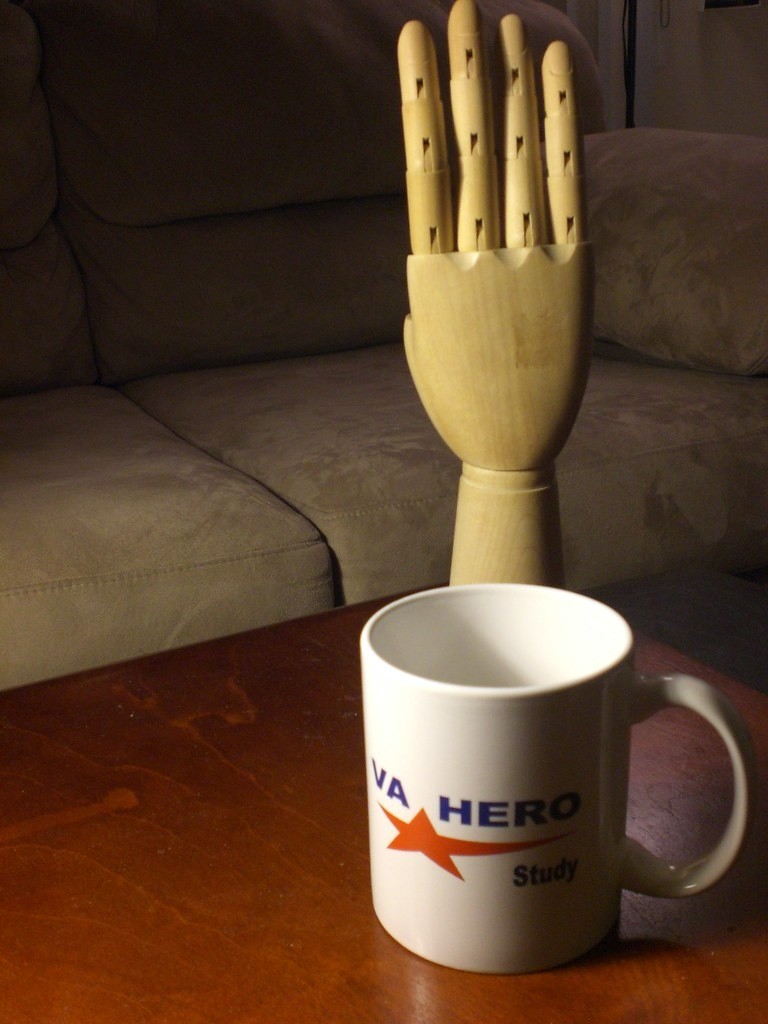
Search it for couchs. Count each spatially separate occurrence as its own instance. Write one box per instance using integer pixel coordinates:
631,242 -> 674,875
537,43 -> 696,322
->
0,0 -> 768,708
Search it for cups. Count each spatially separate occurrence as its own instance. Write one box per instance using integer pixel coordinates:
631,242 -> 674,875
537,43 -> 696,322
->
359,584 -> 749,974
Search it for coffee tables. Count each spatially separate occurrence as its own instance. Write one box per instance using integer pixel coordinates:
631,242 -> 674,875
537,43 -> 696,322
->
0,584 -> 768,1024
